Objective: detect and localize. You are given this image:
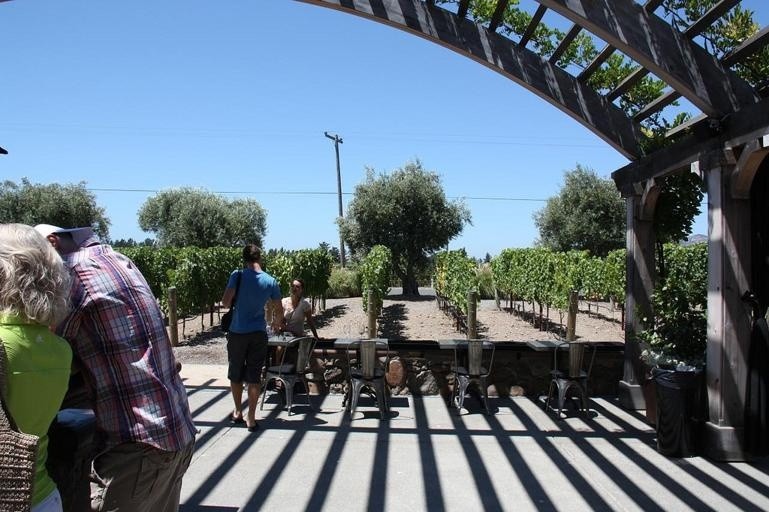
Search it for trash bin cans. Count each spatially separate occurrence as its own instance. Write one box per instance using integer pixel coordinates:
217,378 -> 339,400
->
653,364 -> 707,458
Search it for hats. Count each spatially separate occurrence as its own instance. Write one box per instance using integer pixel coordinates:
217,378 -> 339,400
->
33,222 -> 95,247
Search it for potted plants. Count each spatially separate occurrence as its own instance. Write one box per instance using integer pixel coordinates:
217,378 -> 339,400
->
627,283 -> 711,457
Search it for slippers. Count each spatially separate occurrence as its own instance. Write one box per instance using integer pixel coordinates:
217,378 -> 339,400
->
227,410 -> 243,425
245,420 -> 259,433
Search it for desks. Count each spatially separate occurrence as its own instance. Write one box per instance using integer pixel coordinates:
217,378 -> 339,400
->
332,337 -> 391,408
264,331 -> 298,406
436,336 -> 495,411
526,336 -> 590,419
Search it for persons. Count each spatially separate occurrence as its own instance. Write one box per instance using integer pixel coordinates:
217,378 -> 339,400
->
222,244 -> 284,431
0,223 -> 74,512
33,224 -> 198,512
275,279 -> 324,390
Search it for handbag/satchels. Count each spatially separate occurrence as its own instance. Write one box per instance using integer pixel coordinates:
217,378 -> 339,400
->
220,312 -> 234,331
0,396 -> 42,512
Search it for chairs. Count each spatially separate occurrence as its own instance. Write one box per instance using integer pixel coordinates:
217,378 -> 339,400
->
259,335 -> 320,416
545,335 -> 593,422
450,338 -> 495,418
344,338 -> 392,423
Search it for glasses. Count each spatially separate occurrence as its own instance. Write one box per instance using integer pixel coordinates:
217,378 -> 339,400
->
290,284 -> 302,288
